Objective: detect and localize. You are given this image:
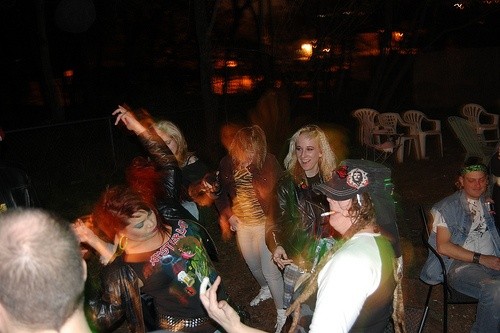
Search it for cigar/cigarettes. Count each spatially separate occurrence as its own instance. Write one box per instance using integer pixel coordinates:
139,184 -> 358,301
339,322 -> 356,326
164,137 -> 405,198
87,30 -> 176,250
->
320,211 -> 335,217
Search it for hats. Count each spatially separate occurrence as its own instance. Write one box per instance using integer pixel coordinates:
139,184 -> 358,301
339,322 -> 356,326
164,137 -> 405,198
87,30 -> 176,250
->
312,158 -> 394,201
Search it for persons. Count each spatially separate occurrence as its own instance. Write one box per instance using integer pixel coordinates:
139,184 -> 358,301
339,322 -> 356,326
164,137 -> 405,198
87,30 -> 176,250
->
0,205 -> 94,332
419,160 -> 500,333
198,158 -> 410,333
66,104 -> 339,333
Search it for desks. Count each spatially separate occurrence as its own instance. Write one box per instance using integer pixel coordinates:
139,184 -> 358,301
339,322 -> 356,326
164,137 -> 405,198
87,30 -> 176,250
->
373,131 -> 404,166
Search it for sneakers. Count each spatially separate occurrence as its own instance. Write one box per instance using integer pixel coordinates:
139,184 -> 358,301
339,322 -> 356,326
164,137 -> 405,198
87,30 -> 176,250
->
273,305 -> 288,333
250,286 -> 272,306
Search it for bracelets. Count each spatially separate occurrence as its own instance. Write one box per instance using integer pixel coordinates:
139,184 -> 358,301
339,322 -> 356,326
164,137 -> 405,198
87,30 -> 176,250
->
473,251 -> 481,265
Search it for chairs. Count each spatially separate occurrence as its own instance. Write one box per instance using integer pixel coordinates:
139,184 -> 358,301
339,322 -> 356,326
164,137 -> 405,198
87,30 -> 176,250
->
352,107 -> 444,167
405,200 -> 500,333
447,104 -> 500,176
